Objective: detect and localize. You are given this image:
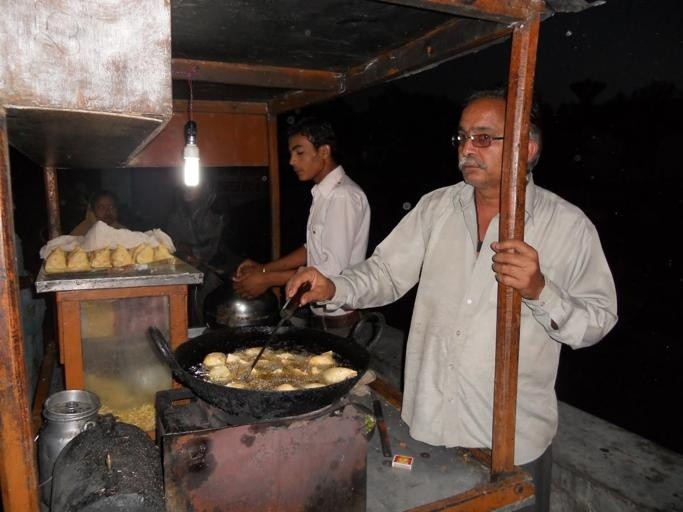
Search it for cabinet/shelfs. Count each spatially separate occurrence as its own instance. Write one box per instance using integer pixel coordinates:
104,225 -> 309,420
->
35,258 -> 207,443
31,321 -> 538,511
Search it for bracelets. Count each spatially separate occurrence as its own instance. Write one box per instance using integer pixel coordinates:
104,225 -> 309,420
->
260,261 -> 270,275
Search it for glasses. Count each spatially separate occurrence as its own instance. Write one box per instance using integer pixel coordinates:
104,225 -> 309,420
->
449,132 -> 534,146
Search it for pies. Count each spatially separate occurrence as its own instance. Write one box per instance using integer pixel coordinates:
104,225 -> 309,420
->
45,242 -> 172,274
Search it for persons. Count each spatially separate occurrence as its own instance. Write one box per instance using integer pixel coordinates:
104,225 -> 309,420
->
153,161 -> 228,319
73,190 -> 140,239
283,87 -> 622,512
229,114 -> 372,361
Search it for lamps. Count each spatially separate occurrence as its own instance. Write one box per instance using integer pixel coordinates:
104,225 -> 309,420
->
182,72 -> 203,189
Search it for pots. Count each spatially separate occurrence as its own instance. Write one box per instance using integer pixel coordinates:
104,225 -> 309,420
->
202,277 -> 281,327
146,310 -> 393,421
